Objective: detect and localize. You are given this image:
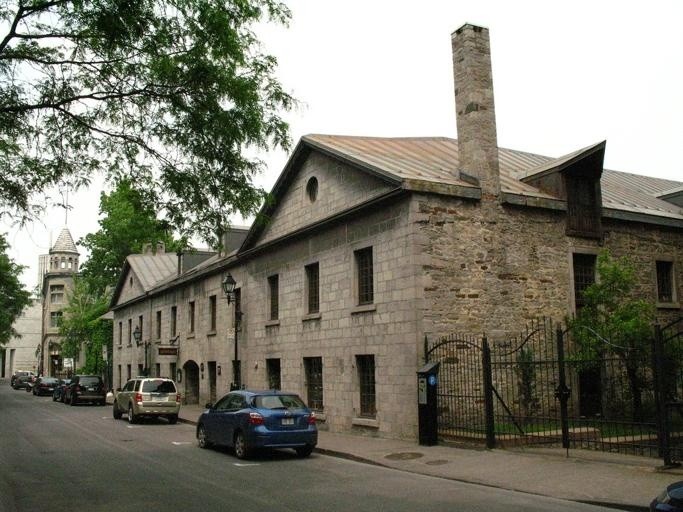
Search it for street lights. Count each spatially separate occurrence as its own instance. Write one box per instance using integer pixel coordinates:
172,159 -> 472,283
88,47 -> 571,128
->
86,339 -> 100,375
222,272 -> 243,391
132,325 -> 150,377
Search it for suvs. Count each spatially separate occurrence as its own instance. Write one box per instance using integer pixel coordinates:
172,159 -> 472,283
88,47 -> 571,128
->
111,375 -> 184,424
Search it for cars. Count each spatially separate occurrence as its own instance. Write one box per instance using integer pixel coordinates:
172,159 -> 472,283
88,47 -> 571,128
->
648,477 -> 683,511
10,369 -> 109,407
194,389 -> 324,461
